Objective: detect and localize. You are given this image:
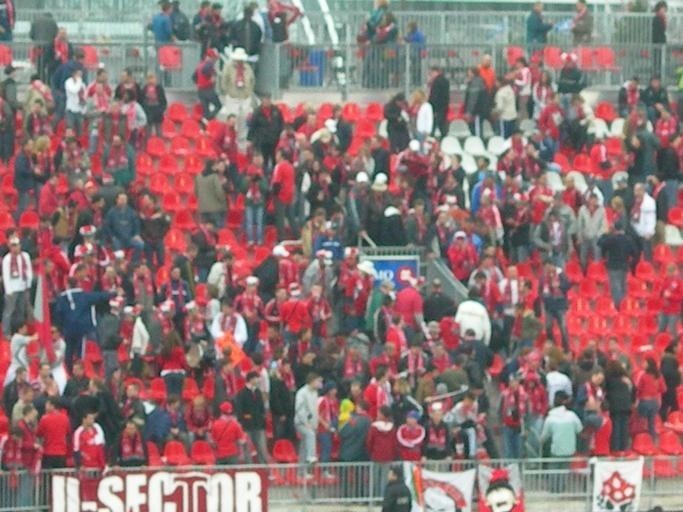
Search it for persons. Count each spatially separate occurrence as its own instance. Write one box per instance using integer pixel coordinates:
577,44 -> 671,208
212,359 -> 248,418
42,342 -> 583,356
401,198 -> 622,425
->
380,466 -> 411,512
1,0 -> 682,497
